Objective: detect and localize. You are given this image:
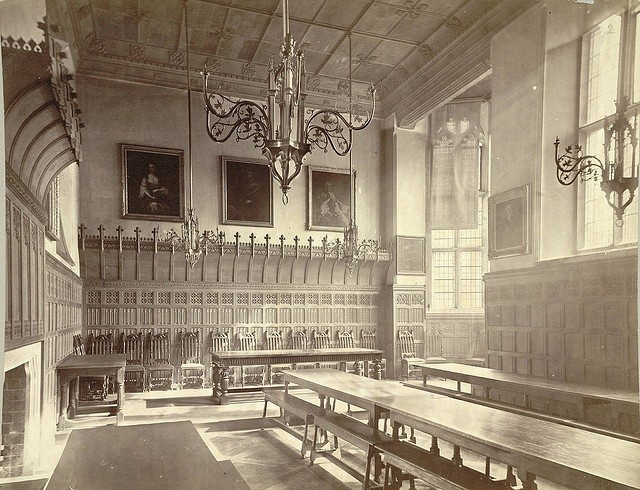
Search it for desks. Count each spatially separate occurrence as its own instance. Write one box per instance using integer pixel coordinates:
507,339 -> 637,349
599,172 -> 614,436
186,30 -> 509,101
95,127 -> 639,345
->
413,361 -> 462,400
377,396 -> 640,490
282,367 -> 446,432
57,353 -> 125,426
210,348 -> 383,406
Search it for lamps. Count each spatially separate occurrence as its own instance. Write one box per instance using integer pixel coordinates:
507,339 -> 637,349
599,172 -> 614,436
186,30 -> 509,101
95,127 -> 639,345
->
154,204 -> 225,268
201,0 -> 376,205
553,96 -> 640,229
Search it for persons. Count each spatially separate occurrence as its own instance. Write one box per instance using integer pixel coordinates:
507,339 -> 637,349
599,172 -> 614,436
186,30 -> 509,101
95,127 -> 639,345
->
442,148 -> 474,223
446,118 -> 456,136
460,117 -> 474,133
498,204 -> 522,248
136,160 -> 175,216
315,179 -> 350,227
232,169 -> 268,221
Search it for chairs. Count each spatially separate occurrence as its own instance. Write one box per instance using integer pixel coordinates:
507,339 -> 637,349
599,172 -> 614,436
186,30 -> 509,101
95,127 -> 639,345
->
337,329 -> 364,376
179,331 -> 204,390
72,334 -> 108,400
361,330 -> 387,381
147,332 -> 174,389
314,330 -> 334,368
266,331 -> 286,383
424,330 -> 446,381
90,333 -> 115,394
120,332 -> 145,392
211,331 -> 235,387
465,330 -> 486,368
290,330 -> 312,369
397,330 -> 427,382
239,331 -> 265,387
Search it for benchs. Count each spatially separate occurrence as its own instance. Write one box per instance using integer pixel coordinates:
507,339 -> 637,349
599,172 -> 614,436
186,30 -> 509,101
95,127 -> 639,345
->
310,404 -> 398,490
400,378 -> 468,400
257,387 -> 329,458
567,419 -> 636,441
374,440 -> 494,489
464,396 -> 571,424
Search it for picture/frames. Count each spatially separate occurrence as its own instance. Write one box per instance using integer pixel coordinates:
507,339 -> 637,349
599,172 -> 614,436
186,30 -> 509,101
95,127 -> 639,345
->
120,143 -> 185,222
395,234 -> 426,275
221,155 -> 275,228
488,185 -> 531,259
307,164 -> 357,233
